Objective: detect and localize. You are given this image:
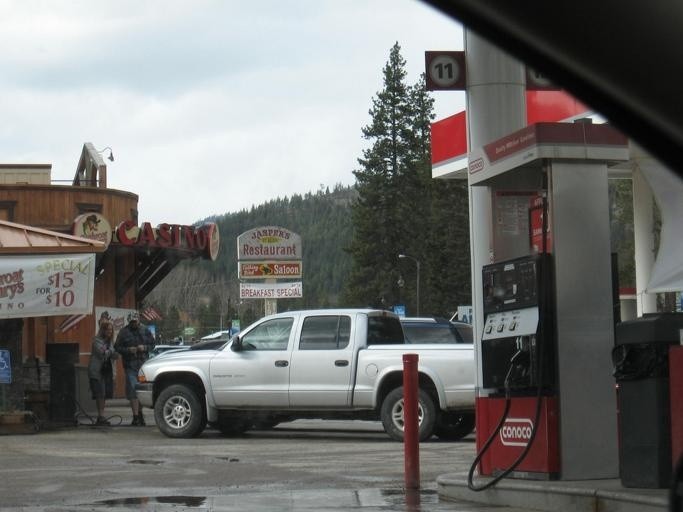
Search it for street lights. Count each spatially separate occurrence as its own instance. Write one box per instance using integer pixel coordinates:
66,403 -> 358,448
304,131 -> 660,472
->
399,254 -> 420,316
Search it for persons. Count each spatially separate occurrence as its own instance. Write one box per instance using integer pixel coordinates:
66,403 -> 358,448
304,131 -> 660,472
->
114,311 -> 155,429
89,323 -> 120,426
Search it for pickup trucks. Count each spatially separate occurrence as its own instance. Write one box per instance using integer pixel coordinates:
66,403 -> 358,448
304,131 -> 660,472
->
134,303 -> 474,442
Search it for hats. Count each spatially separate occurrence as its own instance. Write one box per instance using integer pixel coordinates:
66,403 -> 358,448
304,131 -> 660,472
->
127,310 -> 139,322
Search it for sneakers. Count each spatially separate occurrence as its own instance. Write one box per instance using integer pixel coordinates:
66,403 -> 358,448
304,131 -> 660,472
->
97,416 -> 110,425
132,419 -> 146,427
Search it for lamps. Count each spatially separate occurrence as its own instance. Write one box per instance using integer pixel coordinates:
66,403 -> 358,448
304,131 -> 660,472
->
98,146 -> 115,162
